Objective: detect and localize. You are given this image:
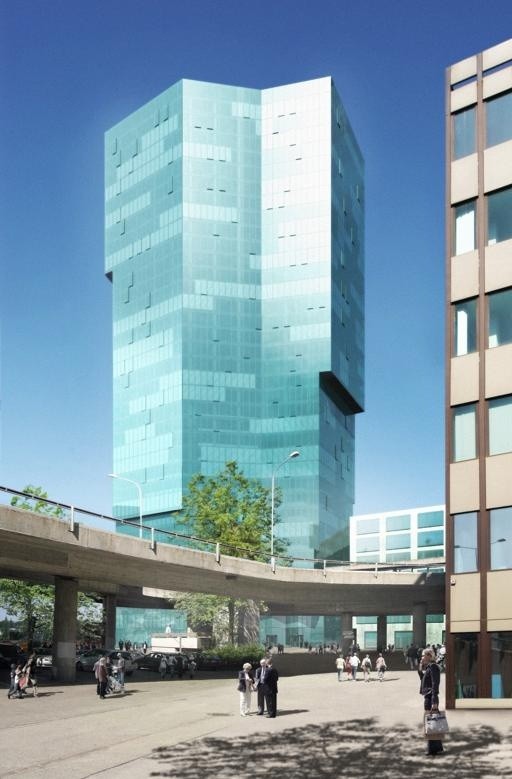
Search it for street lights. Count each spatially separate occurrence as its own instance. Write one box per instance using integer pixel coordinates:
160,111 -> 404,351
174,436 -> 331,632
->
108,473 -> 143,537
269,450 -> 301,557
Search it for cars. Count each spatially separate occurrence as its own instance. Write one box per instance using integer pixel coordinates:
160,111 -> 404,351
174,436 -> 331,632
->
29,647 -> 53,670
1,640 -> 28,672
239,655 -> 261,671
190,652 -> 222,671
76,647 -> 133,677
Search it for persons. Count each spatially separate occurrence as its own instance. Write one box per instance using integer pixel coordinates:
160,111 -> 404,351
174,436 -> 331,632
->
418,648 -> 443,756
238,642 -> 283,718
8,623 -> 196,700
299,639 -> 446,682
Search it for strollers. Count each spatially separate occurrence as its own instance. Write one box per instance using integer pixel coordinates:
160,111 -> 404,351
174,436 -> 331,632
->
105,666 -> 126,694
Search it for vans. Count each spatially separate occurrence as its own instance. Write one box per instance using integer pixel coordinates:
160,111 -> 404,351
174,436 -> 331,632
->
133,651 -> 189,673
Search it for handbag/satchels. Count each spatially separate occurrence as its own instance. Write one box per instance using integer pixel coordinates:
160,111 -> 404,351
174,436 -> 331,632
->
424,708 -> 450,740
